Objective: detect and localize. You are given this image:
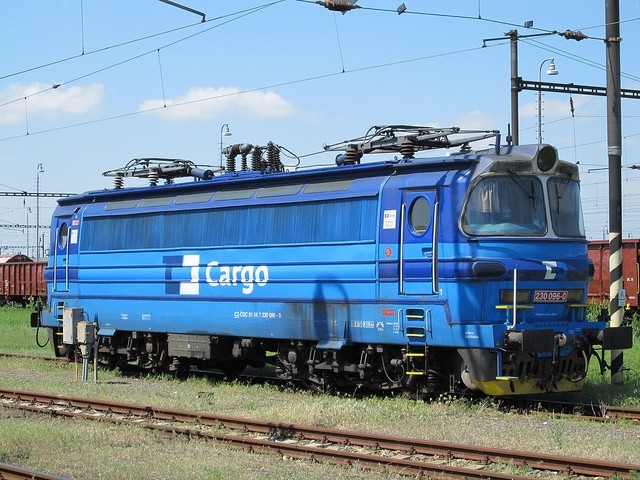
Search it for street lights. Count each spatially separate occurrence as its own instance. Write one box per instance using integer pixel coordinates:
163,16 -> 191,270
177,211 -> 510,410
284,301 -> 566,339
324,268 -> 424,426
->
535,58 -> 558,144
36,163 -> 44,263
27,207 -> 32,259
217,124 -> 232,175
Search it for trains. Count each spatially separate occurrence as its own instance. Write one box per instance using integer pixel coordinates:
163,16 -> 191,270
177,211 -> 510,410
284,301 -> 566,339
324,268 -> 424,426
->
31,125 -> 605,400
4,238 -> 639,307
